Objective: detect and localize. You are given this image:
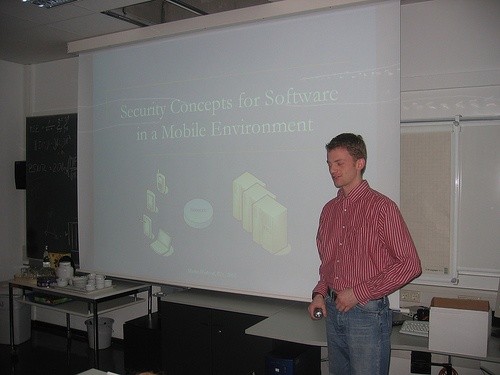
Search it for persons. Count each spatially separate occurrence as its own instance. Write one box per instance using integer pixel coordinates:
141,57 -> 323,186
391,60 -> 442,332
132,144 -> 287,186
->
307,131 -> 422,375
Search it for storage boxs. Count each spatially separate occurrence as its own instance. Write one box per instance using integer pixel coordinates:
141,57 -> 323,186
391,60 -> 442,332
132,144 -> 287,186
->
427,297 -> 493,357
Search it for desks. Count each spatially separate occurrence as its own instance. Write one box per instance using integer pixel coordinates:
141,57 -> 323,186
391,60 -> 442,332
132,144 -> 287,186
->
244,301 -> 500,375
7,276 -> 153,367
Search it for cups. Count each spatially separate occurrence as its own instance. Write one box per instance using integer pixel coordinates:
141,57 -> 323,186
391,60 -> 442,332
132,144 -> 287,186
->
456,295 -> 468,308
412,308 -> 429,321
469,296 -> 479,310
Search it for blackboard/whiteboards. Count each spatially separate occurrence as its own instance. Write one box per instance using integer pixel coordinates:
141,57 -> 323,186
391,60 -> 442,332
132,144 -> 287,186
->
456,113 -> 499,280
24,111 -> 78,266
400,120 -> 458,283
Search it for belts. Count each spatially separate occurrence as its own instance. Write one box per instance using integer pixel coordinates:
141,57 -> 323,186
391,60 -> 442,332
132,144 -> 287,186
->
327,287 -> 337,302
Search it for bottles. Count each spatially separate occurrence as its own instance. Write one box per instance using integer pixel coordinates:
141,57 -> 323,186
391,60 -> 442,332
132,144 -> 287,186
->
42,246 -> 51,270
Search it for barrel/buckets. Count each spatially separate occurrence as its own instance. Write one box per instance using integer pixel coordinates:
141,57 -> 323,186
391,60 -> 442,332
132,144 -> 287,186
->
84,317 -> 114,350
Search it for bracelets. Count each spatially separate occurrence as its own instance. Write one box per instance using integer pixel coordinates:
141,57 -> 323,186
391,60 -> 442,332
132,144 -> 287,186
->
312,292 -> 325,299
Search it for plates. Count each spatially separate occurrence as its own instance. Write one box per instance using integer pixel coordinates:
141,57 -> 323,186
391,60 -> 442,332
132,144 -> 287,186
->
104,280 -> 112,288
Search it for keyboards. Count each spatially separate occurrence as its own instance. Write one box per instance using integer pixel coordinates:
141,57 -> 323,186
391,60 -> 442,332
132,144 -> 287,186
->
399,320 -> 429,337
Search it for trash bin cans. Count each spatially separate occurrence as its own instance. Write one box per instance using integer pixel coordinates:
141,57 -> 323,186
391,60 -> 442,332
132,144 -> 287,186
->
83,317 -> 115,350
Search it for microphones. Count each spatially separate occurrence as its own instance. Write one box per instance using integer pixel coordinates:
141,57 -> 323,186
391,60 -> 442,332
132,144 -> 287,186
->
314,309 -> 323,318
